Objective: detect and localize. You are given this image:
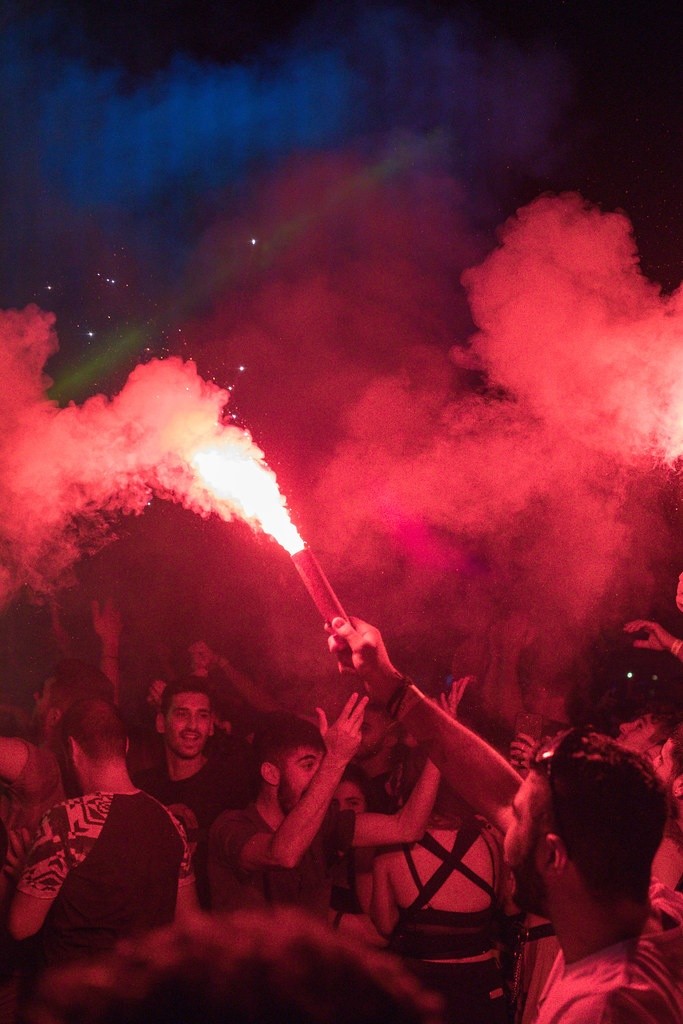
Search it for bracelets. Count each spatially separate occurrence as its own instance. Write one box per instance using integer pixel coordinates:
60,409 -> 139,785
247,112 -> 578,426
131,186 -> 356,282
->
385,675 -> 414,719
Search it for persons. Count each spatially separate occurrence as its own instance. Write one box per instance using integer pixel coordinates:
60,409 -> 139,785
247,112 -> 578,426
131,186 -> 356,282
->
0,582 -> 471,1024
322,567 -> 681,1024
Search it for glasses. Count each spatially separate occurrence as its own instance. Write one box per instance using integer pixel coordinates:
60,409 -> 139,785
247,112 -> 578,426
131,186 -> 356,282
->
536,723 -> 587,861
